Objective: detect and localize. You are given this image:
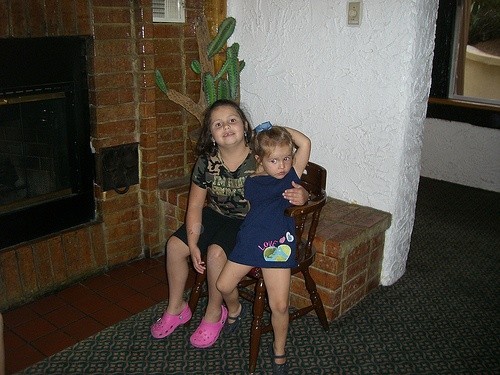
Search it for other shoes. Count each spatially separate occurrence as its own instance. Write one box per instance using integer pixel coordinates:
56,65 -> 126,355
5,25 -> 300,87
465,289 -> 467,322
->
223,304 -> 247,337
268,343 -> 289,375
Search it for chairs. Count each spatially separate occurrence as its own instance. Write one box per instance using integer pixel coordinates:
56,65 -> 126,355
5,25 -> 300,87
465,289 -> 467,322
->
185,161 -> 330,375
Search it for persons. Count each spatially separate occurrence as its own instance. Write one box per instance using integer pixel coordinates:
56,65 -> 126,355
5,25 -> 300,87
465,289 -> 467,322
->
150,98 -> 310,348
216,121 -> 311,375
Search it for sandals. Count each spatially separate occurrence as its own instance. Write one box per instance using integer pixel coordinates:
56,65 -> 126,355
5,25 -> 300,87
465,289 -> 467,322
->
190,305 -> 227,348
151,303 -> 192,338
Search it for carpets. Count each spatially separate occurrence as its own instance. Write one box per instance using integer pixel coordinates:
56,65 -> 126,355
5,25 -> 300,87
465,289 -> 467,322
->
15,176 -> 500,375
